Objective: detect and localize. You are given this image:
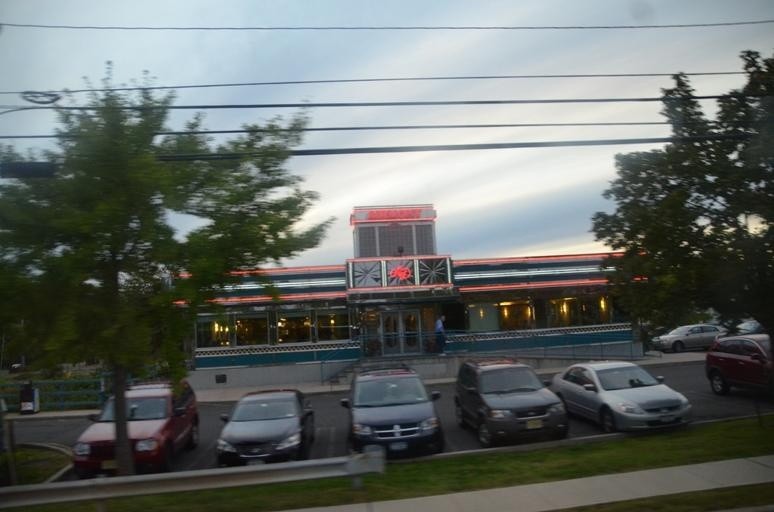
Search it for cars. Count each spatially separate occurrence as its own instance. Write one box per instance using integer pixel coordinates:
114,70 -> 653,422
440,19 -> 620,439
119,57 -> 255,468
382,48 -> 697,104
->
217,390 -> 313,466
341,368 -> 444,452
455,357 -> 569,447
652,320 -> 760,352
706,334 -> 774,394
73,379 -> 199,478
552,360 -> 693,431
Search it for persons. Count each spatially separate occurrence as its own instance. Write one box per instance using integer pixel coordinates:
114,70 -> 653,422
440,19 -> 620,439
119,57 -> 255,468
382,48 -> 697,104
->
432,315 -> 449,355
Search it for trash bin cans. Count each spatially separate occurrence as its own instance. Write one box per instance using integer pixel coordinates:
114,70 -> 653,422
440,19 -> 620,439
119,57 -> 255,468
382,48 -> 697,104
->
19,389 -> 40,415
